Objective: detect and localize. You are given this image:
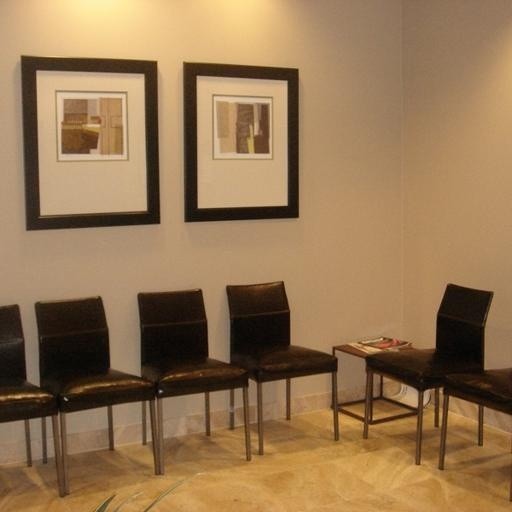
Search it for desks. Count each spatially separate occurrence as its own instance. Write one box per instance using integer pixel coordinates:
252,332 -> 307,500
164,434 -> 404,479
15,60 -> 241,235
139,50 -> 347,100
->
332,340 -> 419,424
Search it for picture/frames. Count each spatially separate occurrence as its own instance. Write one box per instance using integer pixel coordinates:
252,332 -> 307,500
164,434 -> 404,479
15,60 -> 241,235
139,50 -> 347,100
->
181,59 -> 301,224
21,54 -> 162,231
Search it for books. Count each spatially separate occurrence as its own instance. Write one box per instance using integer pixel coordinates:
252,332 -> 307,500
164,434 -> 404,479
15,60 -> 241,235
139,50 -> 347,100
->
358,336 -> 411,349
347,339 -> 398,354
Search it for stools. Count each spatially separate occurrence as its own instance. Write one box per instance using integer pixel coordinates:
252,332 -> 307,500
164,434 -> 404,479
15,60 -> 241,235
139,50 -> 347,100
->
436,368 -> 512,470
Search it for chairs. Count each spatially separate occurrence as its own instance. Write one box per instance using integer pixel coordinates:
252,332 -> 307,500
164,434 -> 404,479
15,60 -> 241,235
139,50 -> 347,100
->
227,281 -> 339,454
363,283 -> 495,466
137,289 -> 252,474
35,295 -> 160,494
2,304 -> 64,498
